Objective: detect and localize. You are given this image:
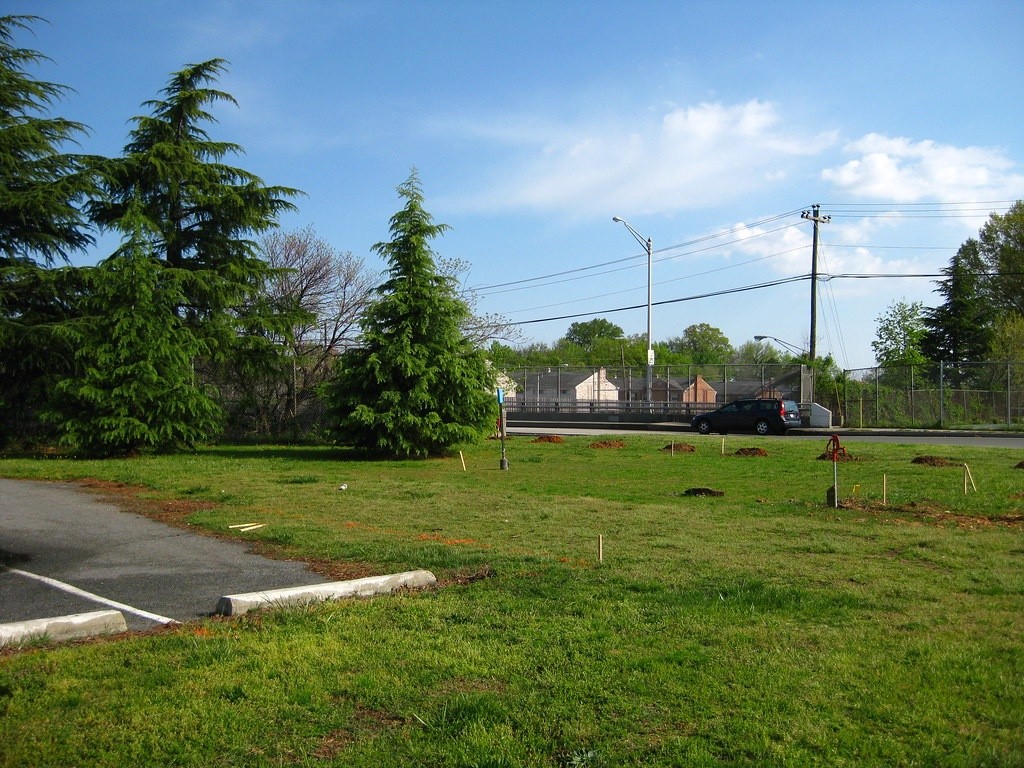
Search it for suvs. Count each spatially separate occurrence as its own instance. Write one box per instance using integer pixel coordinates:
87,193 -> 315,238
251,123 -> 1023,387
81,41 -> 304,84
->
689,395 -> 802,436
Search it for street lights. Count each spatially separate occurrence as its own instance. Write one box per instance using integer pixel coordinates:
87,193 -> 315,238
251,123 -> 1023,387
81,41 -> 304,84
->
612,216 -> 652,401
753,334 -> 817,402
535,367 -> 551,413
